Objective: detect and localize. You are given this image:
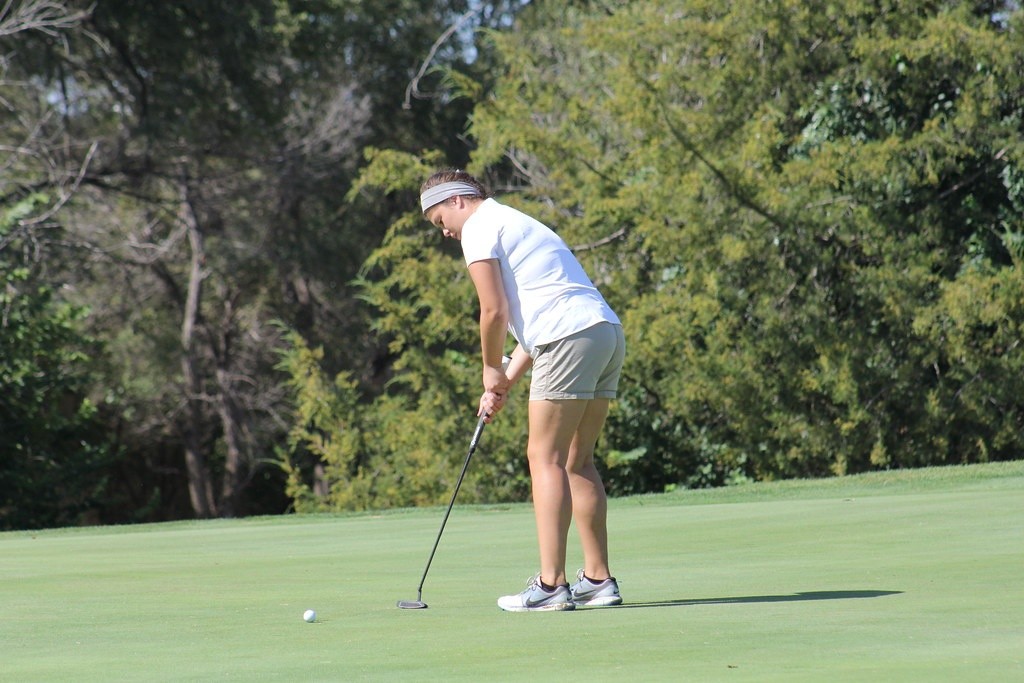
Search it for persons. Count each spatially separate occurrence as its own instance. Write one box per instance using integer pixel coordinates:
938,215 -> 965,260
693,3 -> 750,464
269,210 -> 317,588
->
419,168 -> 627,616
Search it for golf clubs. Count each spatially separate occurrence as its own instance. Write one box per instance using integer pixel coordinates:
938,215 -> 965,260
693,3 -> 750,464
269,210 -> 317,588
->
396,353 -> 513,608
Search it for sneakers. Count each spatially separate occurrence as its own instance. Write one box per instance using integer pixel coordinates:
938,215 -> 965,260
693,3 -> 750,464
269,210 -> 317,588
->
564,572 -> 622,606
496,576 -> 578,612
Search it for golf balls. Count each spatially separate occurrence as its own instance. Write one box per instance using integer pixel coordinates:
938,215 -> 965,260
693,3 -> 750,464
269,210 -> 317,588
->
303,609 -> 316,623
